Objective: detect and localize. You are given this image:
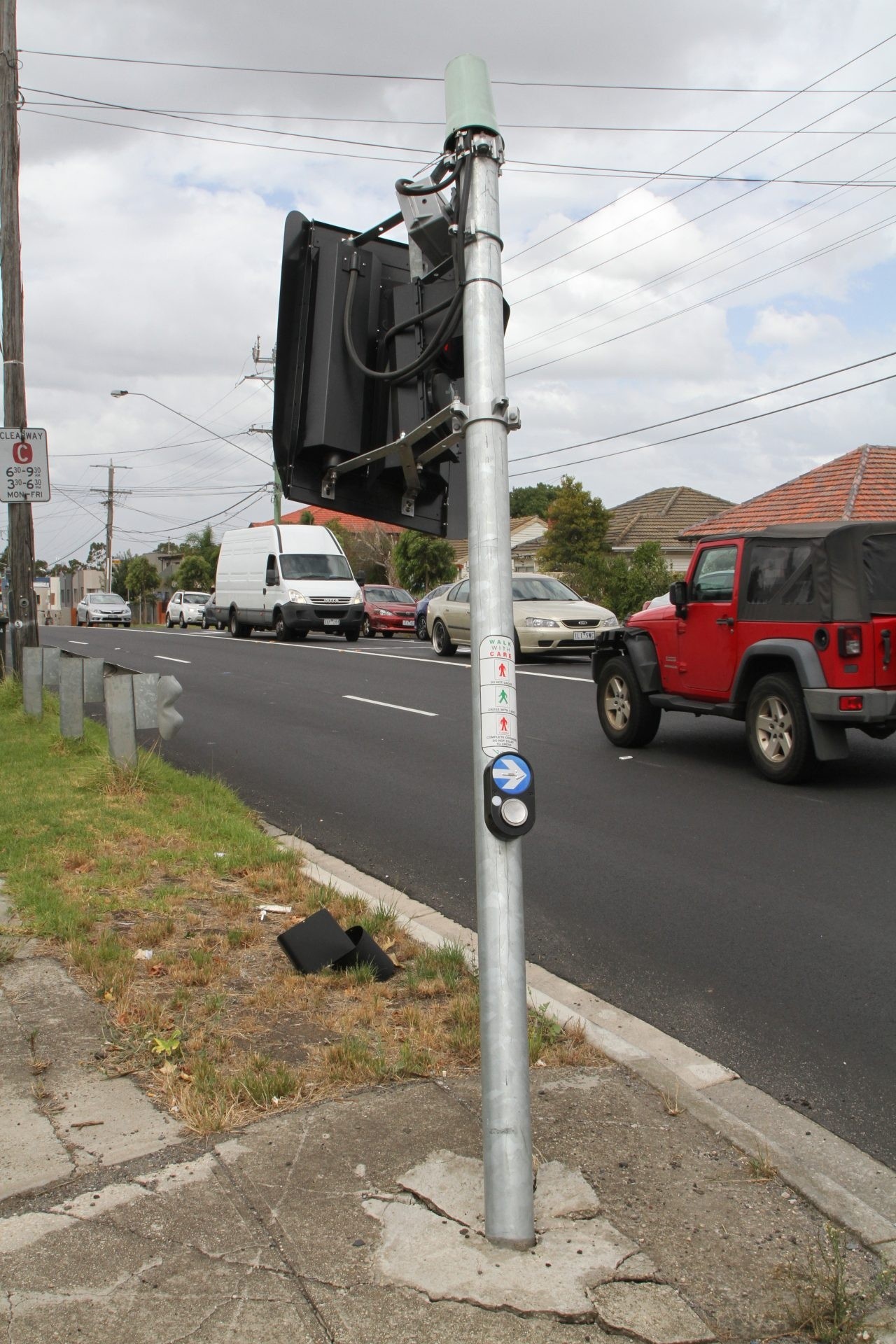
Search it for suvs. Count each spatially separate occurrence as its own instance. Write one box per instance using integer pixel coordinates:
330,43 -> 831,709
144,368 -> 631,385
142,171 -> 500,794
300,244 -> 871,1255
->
166,589 -> 225,630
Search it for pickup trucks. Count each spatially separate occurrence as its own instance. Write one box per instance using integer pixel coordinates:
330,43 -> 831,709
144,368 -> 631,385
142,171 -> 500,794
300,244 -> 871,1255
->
589,522 -> 896,783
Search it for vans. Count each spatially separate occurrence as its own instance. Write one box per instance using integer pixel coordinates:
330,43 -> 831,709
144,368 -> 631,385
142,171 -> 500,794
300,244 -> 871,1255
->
216,524 -> 365,643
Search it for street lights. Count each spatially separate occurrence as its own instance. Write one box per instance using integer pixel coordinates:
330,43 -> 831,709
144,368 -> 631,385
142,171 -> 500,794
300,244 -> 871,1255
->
110,391 -> 281,525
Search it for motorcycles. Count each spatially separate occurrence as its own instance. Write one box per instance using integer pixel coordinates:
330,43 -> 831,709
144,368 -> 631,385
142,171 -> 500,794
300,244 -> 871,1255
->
44,617 -> 51,625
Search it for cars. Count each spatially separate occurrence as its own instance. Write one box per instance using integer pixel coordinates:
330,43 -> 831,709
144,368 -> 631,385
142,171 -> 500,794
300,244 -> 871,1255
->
76,593 -> 131,627
361,573 -> 621,664
